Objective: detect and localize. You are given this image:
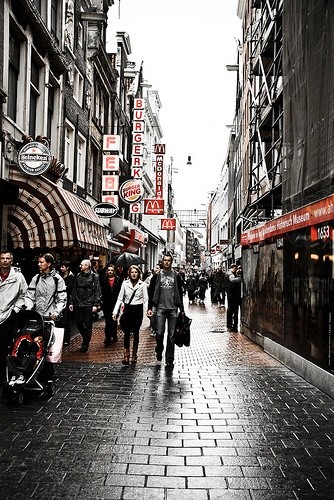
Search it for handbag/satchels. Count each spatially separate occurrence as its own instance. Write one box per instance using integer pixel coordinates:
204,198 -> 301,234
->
172,312 -> 192,347
120,311 -> 129,332
44,320 -> 64,363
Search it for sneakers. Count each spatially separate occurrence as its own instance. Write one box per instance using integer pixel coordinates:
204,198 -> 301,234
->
9,375 -> 17,386
16,373 -> 25,384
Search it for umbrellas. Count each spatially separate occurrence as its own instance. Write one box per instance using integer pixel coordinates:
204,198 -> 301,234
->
107,251 -> 147,266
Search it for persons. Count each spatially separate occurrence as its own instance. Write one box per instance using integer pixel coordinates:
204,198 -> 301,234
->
112,265 -> 148,364
25,253 -> 68,382
147,254 -> 184,368
60,258 -> 243,352
0,251 -> 28,377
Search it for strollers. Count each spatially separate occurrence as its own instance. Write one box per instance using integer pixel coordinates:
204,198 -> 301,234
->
0,307 -> 55,406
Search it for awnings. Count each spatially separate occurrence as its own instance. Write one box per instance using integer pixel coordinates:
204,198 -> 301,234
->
7,168 -> 108,255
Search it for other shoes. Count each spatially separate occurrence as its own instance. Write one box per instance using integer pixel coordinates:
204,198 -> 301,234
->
104,339 -> 111,344
156,349 -> 162,361
63,339 -> 70,344
233,329 -> 238,332
113,336 -> 118,343
167,362 -> 174,368
81,345 -> 88,353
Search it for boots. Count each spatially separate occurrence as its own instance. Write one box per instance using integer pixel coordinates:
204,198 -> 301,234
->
132,349 -> 137,363
122,349 -> 130,365
